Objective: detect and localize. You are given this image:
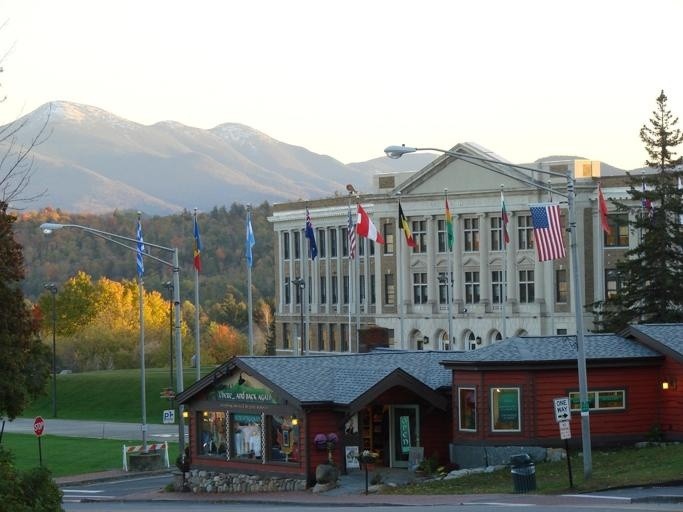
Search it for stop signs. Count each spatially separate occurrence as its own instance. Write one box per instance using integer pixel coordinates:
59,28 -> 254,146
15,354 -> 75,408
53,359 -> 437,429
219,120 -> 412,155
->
34,417 -> 46,438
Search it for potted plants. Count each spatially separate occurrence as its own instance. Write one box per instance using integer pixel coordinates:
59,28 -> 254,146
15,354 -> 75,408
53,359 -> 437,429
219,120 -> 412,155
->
359,447 -> 380,472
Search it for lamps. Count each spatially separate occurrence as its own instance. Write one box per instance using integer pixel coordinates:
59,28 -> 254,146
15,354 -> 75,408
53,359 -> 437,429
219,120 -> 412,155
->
291,414 -> 304,430
658,376 -> 677,391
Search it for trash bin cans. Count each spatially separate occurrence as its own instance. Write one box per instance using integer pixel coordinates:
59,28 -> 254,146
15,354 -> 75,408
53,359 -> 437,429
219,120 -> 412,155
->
510,454 -> 536,494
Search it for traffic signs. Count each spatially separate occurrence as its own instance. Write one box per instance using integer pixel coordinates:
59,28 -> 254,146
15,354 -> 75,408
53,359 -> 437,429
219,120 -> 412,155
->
554,396 -> 573,424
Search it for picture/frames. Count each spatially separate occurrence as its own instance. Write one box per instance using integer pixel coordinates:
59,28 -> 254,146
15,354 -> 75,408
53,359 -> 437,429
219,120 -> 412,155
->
281,429 -> 290,448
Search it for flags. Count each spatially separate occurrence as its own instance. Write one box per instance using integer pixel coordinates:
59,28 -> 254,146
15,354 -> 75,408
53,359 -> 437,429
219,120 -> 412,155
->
354,195 -> 385,245
443,188 -> 454,251
134,209 -> 146,281
500,184 -> 510,246
526,202 -> 567,262
397,191 -> 417,249
345,207 -> 356,262
642,175 -> 655,218
303,204 -> 319,262
597,180 -> 611,237
243,201 -> 255,270
191,204 -> 202,278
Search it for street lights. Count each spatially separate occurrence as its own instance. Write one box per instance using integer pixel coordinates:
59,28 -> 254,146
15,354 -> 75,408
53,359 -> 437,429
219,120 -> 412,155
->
292,276 -> 308,356
384,141 -> 596,485
44,281 -> 59,419
39,220 -> 189,472
162,279 -> 177,411
437,269 -> 453,352
